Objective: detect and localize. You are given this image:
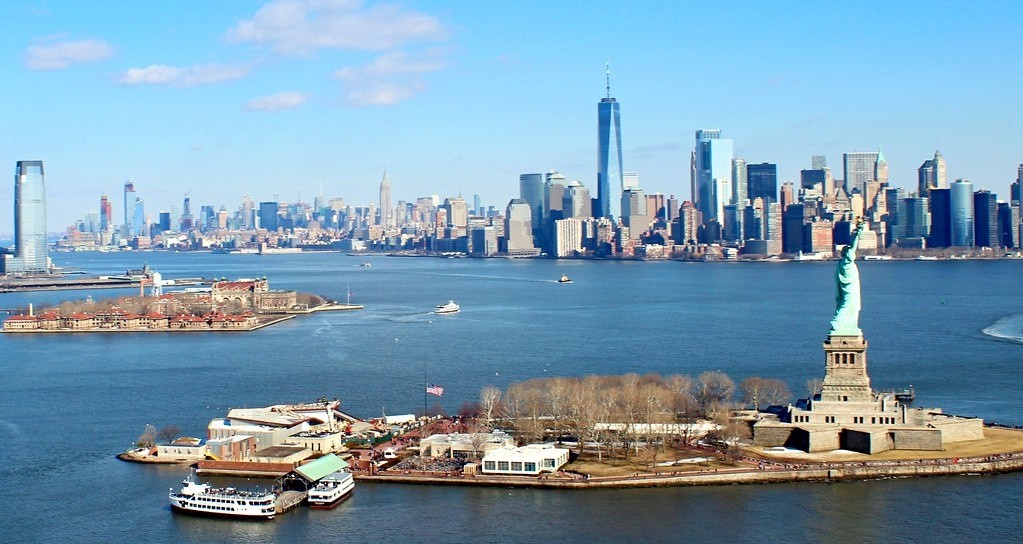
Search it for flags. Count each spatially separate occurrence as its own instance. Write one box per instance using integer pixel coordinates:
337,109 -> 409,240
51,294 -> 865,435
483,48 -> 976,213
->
427,383 -> 443,396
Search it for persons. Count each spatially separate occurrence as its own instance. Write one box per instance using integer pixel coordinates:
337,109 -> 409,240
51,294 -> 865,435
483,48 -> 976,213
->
988,452 -> 1012,461
371,421 -> 443,453
827,227 -> 863,335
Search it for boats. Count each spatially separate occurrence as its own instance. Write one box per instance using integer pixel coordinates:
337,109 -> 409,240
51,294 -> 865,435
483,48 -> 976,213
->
360,263 -> 372,267
169,479 -> 276,519
435,299 -> 461,314
557,275 -> 572,284
307,473 -> 355,510
